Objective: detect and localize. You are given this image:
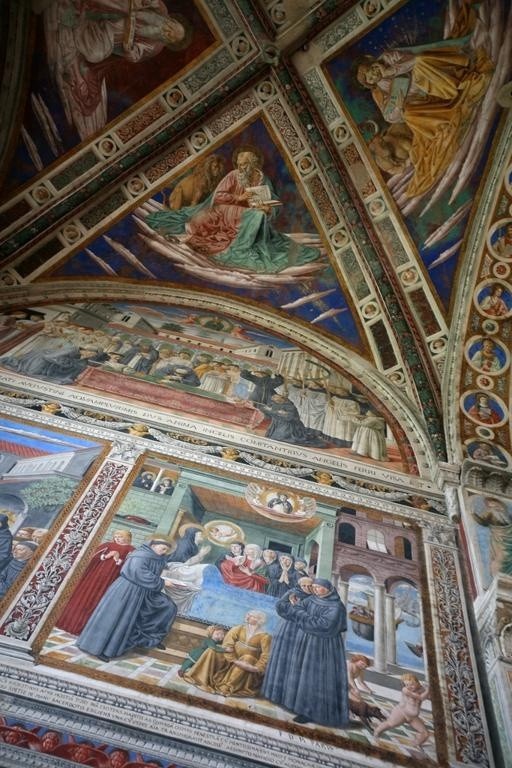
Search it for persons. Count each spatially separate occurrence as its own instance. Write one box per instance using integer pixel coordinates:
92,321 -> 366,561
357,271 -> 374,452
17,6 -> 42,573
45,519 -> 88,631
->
465,494 -> 512,578
54,0 -> 187,64
190,151 -> 281,250
354,45 -> 471,140
456,215 -> 512,469
1,304 -> 446,758
